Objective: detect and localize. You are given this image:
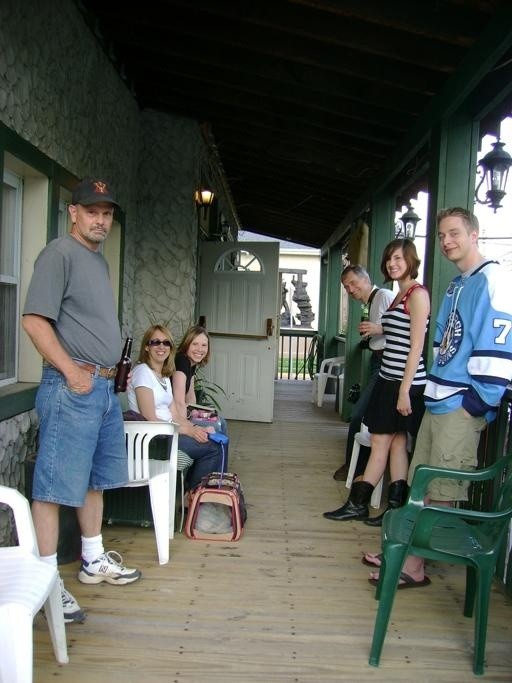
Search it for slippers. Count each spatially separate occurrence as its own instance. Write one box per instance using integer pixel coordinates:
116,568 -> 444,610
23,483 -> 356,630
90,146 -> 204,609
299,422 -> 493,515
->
361,551 -> 386,569
368,567 -> 432,590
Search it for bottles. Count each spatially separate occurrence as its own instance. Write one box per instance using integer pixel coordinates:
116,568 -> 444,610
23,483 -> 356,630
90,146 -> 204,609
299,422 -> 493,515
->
113,337 -> 134,394
359,300 -> 370,336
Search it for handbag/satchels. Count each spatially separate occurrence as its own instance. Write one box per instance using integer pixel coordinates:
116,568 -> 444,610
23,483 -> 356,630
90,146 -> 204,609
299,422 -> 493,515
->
188,468 -> 247,543
186,402 -> 218,421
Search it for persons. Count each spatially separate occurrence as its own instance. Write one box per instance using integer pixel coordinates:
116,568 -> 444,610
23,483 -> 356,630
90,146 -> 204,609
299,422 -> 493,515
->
362,206 -> 512,591
21,177 -> 143,624
333,263 -> 396,482
171,325 -> 228,433
126,324 -> 223,514
322,238 -> 431,528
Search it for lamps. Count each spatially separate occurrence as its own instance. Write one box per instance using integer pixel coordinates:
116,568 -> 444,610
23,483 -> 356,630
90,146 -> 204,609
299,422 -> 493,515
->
476,139 -> 512,214
395,204 -> 422,242
195,183 -> 215,208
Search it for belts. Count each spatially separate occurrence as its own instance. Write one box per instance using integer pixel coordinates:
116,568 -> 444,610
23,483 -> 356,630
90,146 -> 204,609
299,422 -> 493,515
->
79,364 -> 120,379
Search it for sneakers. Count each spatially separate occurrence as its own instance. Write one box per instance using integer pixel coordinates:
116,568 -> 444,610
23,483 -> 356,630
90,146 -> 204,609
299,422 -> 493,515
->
76,550 -> 142,587
37,572 -> 86,625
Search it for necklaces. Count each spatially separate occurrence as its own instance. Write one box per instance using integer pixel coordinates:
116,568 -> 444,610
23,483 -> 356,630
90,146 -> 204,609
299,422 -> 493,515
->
152,369 -> 168,387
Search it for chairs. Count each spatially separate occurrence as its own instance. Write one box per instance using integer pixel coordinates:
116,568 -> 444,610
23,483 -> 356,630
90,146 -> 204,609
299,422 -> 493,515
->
345,421 -> 414,509
369,447 -> 512,676
124,420 -> 181,564
0,485 -> 70,683
311,356 -> 345,415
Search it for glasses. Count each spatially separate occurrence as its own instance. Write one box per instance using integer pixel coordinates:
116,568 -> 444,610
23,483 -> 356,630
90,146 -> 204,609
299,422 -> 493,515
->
147,339 -> 171,347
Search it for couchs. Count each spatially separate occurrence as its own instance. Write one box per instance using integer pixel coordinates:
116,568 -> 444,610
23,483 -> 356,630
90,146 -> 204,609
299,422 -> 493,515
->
101,393 -> 194,528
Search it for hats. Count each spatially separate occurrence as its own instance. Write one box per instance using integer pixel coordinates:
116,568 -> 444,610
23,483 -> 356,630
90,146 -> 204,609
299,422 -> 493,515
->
70,176 -> 124,212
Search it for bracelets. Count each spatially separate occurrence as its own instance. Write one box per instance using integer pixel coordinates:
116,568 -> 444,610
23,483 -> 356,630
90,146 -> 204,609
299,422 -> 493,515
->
193,423 -> 198,427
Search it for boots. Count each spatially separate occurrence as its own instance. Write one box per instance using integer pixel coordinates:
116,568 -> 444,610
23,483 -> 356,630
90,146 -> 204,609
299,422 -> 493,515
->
364,479 -> 410,526
323,481 -> 375,520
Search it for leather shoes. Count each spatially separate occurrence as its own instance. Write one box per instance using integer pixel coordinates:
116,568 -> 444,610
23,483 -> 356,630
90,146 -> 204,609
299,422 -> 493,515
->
334,463 -> 348,480
